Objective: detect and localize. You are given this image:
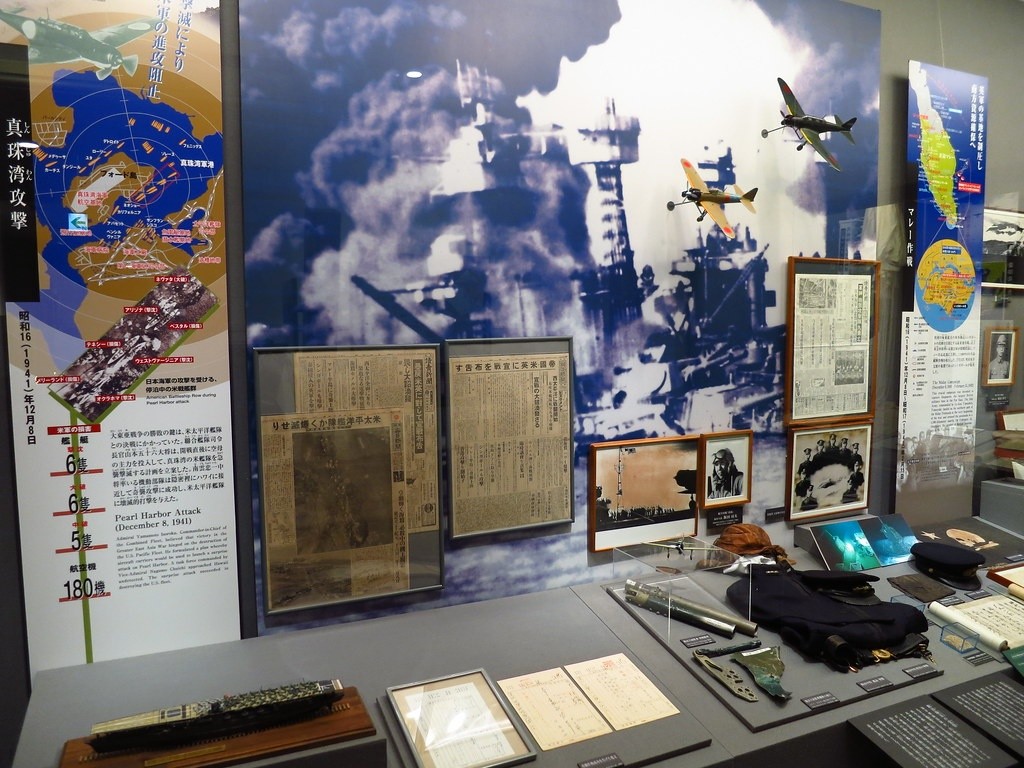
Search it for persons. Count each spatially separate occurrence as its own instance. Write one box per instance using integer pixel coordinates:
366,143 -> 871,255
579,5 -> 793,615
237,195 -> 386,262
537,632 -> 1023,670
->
988,335 -> 1009,381
896,426 -> 974,491
794,433 -> 866,510
708,448 -> 743,499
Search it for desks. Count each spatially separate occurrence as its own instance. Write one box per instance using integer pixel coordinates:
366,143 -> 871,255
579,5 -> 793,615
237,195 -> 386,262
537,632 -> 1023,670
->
16,515 -> 1024,768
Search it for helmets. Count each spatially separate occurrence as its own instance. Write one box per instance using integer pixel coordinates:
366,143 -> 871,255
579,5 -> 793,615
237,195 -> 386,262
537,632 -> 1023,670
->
713,451 -> 734,473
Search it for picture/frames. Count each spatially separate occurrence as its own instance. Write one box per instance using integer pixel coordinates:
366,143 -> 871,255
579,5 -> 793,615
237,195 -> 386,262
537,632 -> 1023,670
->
699,429 -> 754,509
784,255 -> 881,424
251,342 -> 446,617
785,419 -> 873,521
386,666 -> 538,768
443,335 -> 575,541
981,326 -> 1021,388
587,435 -> 701,554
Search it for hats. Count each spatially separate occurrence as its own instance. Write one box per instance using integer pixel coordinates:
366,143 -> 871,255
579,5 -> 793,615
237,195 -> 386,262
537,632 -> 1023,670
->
802,569 -> 882,605
800,434 -> 861,474
910,542 -> 985,590
996,335 -> 1007,345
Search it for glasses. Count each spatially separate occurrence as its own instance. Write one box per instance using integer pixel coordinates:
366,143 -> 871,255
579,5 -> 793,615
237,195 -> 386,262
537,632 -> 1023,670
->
712,450 -> 726,463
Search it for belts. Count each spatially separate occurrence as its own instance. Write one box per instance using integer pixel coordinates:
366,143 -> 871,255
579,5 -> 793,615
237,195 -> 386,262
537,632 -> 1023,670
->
824,632 -> 937,672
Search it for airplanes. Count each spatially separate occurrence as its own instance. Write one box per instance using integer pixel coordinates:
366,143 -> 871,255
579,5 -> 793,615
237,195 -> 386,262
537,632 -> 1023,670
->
761,76 -> 858,173
666,158 -> 758,240
